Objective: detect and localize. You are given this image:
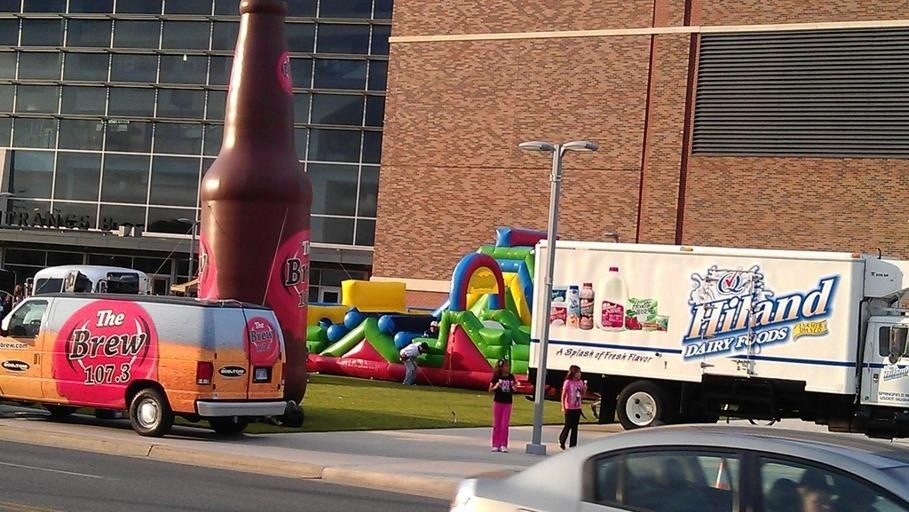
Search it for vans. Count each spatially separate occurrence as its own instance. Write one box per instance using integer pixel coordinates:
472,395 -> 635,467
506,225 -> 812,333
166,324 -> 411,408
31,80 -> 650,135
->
24,260 -> 175,296
2,288 -> 292,439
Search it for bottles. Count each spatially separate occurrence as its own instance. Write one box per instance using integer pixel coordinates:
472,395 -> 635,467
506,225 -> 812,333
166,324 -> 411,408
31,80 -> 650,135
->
191,0 -> 314,407
567,282 -> 595,331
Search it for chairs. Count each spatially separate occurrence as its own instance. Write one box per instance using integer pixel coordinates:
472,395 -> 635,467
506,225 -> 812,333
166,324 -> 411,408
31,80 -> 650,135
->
597,458 -> 797,512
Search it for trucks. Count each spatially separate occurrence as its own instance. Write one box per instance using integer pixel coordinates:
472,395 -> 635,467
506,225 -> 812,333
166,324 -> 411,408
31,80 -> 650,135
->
513,230 -> 909,444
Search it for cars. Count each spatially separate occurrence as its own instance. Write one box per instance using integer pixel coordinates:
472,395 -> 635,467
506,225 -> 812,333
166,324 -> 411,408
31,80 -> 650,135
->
1,290 -> 17,316
434,418 -> 909,512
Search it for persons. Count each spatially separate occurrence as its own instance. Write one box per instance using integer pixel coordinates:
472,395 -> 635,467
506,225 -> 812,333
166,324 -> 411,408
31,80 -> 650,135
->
557,364 -> 588,451
793,485 -> 831,512
424,320 -> 440,337
487,356 -> 520,452
1,282 -> 33,319
399,340 -> 429,386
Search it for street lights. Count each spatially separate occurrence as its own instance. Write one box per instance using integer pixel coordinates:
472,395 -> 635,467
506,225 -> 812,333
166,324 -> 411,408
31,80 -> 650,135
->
517,136 -> 597,459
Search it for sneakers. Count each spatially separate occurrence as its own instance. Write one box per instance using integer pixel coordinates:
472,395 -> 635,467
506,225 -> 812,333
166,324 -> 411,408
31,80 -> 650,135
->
491,446 -> 500,452
501,445 -> 508,452
559,439 -> 565,450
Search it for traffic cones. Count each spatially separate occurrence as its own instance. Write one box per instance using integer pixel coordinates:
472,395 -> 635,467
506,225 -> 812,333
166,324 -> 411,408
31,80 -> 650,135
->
714,457 -> 736,492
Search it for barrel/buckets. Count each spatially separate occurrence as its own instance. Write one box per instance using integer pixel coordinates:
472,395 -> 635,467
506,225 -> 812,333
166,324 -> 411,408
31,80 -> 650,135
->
592,266 -> 630,331
